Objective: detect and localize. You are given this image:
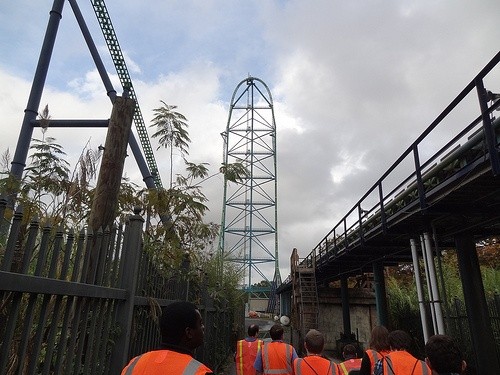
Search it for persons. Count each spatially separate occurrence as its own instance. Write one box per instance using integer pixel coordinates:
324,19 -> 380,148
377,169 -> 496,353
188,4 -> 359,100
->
121,301 -> 215,375
254,324 -> 299,375
336,345 -> 362,375
360,325 -> 467,375
234,324 -> 264,375
294,329 -> 339,375
